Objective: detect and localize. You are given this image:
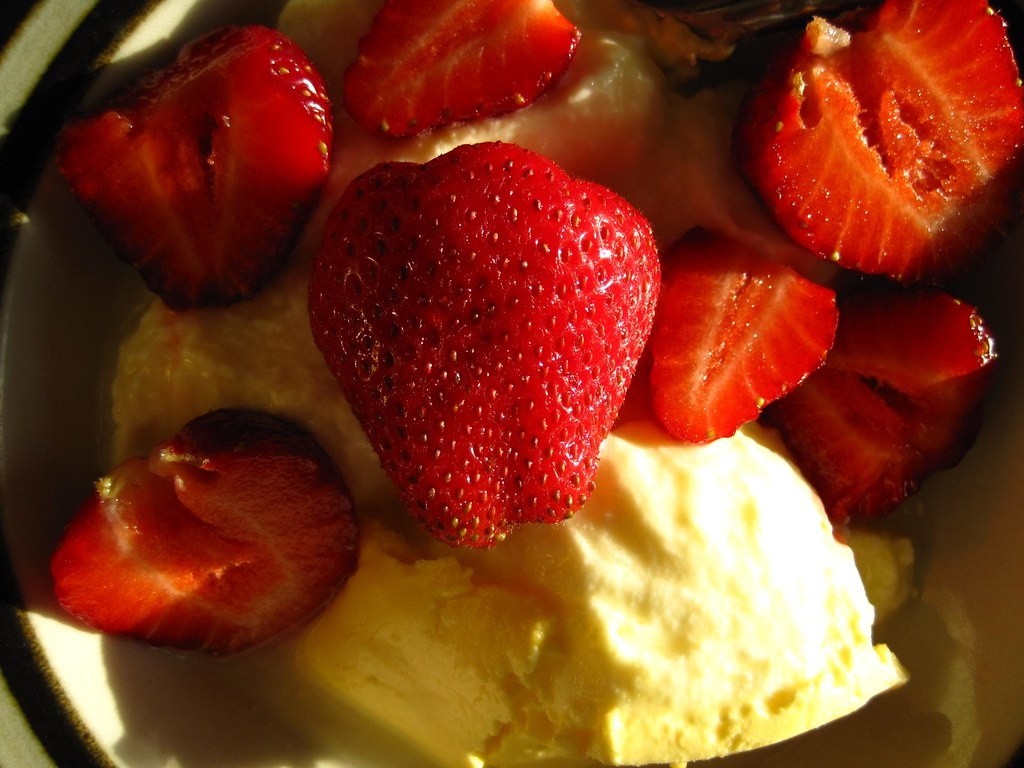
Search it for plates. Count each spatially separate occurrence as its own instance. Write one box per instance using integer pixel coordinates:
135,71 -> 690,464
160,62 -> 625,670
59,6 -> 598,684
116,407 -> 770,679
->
0,0 -> 1024,768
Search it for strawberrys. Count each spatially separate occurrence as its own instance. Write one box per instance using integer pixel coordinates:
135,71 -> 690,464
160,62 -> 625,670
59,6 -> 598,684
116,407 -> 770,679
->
51,0 -> 1024,655
